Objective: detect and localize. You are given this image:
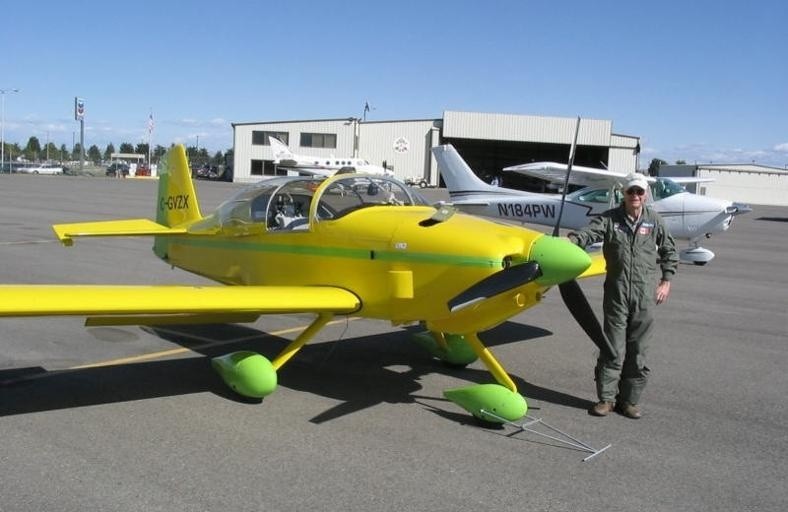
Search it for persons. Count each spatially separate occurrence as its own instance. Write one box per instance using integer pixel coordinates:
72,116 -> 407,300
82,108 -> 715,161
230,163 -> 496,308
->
560,172 -> 680,418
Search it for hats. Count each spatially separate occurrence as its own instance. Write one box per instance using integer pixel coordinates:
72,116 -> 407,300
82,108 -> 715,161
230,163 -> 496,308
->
623,171 -> 649,192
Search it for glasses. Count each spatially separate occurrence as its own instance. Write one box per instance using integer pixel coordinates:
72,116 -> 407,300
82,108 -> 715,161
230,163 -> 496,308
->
626,187 -> 645,195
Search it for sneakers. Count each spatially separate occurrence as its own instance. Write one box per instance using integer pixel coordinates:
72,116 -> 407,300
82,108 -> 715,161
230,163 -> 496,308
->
616,403 -> 641,419
593,401 -> 614,415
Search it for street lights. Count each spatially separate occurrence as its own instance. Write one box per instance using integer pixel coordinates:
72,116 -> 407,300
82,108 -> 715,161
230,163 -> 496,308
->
1,87 -> 20,173
